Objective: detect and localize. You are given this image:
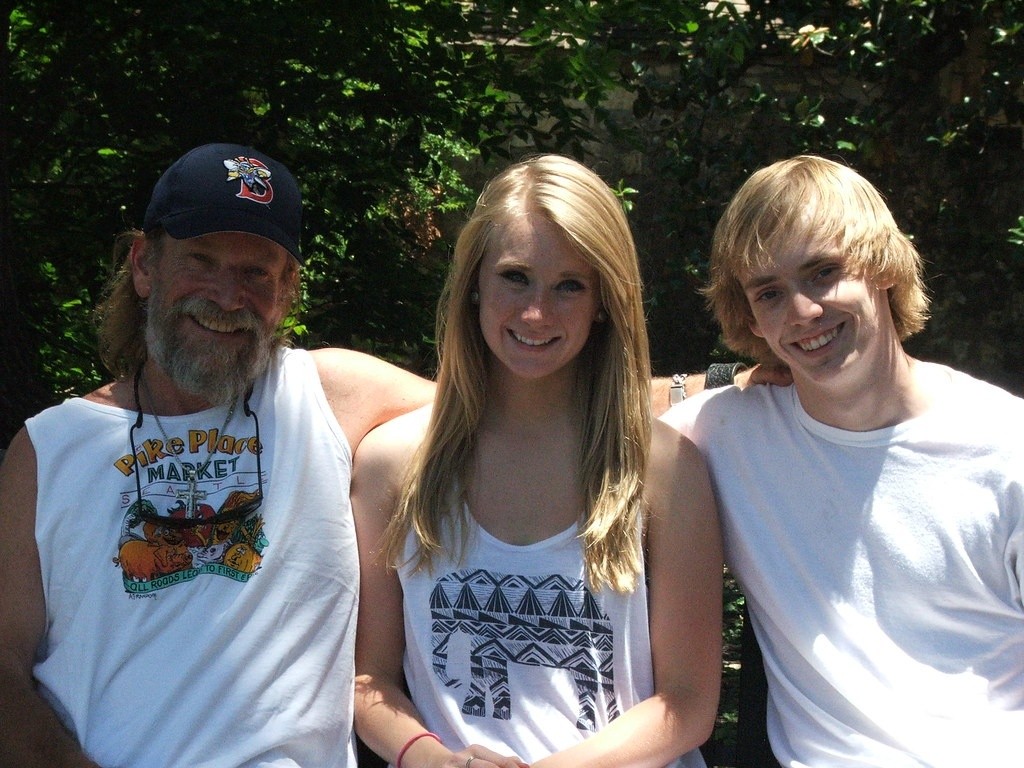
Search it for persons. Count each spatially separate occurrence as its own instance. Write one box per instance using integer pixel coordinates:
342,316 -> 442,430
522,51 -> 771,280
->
0,144 -> 792,768
349,154 -> 724,768
659,152 -> 1024,768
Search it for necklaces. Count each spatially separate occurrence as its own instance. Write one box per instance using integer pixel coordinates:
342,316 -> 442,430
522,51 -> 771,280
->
138,368 -> 243,521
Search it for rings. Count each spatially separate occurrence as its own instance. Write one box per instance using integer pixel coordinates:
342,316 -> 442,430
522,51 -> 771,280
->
465,756 -> 476,768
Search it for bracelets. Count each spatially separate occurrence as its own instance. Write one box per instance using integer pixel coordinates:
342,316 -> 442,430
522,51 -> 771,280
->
669,373 -> 687,409
704,362 -> 749,391
395,733 -> 441,768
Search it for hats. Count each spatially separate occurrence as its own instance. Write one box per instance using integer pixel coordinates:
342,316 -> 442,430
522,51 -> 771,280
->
144,143 -> 305,266
